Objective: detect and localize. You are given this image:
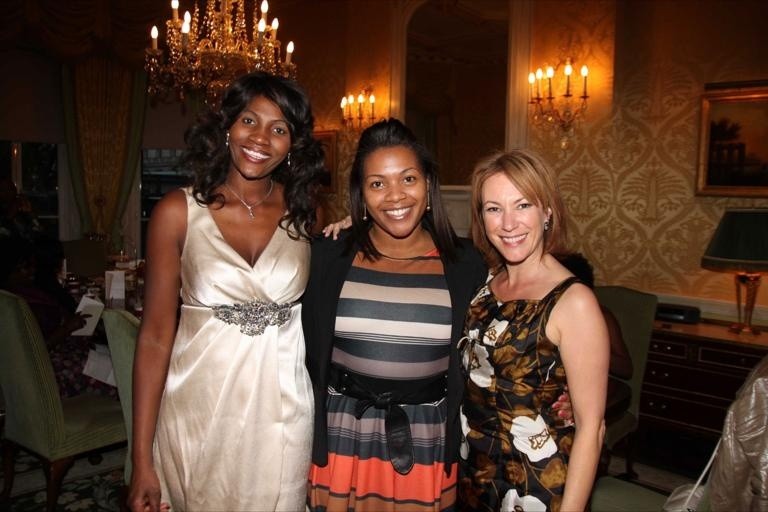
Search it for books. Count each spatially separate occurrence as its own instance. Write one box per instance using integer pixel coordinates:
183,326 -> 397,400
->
71,287 -> 105,337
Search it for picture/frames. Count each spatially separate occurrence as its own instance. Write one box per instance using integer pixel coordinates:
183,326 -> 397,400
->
694,84 -> 768,200
310,127 -> 338,199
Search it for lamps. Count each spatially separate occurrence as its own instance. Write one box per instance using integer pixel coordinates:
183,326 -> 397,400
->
340,89 -> 385,150
527,59 -> 591,152
700,208 -> 768,334
144,1 -> 300,120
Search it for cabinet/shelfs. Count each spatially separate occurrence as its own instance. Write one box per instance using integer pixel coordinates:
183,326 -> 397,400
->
139,174 -> 199,258
636,317 -> 768,484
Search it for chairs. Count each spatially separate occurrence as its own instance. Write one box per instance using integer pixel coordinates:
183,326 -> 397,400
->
99,307 -> 143,486
0,290 -> 125,512
593,284 -> 657,480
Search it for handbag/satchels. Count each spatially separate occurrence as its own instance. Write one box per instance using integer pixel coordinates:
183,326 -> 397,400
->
661,484 -> 705,511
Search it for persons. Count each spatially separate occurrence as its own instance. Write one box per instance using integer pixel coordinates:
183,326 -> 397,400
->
698,347 -> 768,512
127,73 -> 354,512
300,117 -> 486,510
557,246 -> 635,381
321,148 -> 609,511
1,257 -> 92,375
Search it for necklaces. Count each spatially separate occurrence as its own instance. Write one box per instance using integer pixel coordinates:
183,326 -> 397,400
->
224,178 -> 274,222
